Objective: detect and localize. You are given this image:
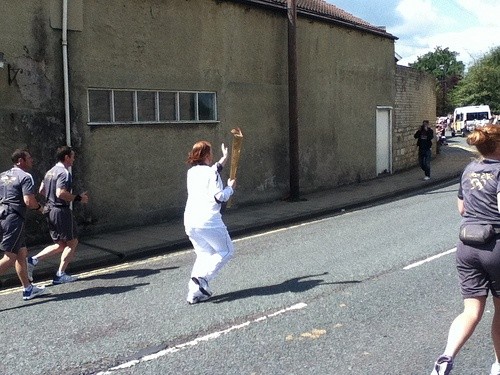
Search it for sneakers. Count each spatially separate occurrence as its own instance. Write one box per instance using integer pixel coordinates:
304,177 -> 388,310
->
25,255 -> 36,282
23,285 -> 46,300
52,270 -> 77,284
192,274 -> 214,296
186,292 -> 212,304
429,354 -> 453,375
490,364 -> 500,375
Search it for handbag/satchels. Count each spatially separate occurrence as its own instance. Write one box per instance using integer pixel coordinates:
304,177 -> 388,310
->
458,223 -> 500,245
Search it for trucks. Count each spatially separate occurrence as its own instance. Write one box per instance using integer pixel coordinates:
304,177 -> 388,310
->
450,105 -> 492,137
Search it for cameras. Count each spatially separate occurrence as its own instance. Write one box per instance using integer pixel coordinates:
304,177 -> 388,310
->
421,126 -> 425,129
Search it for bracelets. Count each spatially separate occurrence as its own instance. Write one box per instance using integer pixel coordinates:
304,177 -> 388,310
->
32,202 -> 42,210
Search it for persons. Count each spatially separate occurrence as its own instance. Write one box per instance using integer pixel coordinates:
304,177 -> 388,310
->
434,110 -> 500,145
431,124 -> 500,375
184,140 -> 238,304
25,146 -> 88,284
413,119 -> 432,181
0,148 -> 46,301
